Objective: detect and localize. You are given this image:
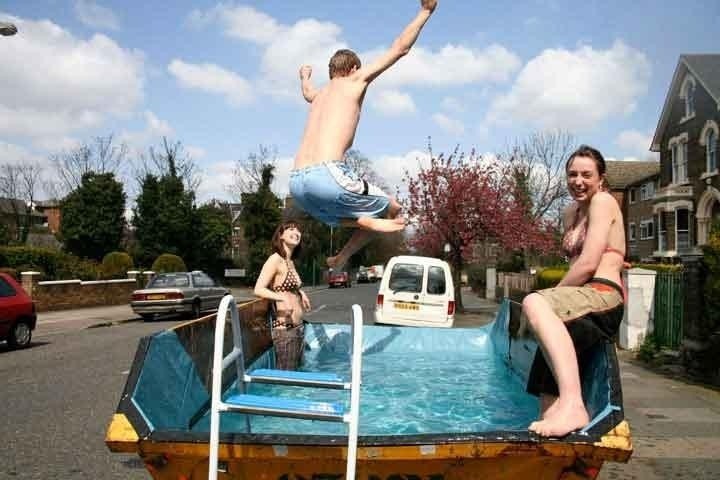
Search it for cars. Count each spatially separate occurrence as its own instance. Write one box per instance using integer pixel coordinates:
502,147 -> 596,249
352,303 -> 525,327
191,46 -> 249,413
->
131,268 -> 234,322
1,271 -> 37,351
326,260 -> 385,289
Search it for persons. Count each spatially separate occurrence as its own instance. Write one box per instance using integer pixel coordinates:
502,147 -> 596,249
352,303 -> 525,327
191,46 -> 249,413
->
522,144 -> 632,438
254,221 -> 311,371
289,0 -> 437,271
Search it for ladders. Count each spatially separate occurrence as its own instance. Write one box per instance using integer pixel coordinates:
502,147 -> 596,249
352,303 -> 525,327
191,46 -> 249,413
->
208,295 -> 363,480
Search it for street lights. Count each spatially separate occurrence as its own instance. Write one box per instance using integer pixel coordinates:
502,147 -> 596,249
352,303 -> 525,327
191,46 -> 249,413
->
1,19 -> 18,37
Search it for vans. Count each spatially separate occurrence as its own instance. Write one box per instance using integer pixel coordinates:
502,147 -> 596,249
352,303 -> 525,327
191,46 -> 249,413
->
372,253 -> 456,330
